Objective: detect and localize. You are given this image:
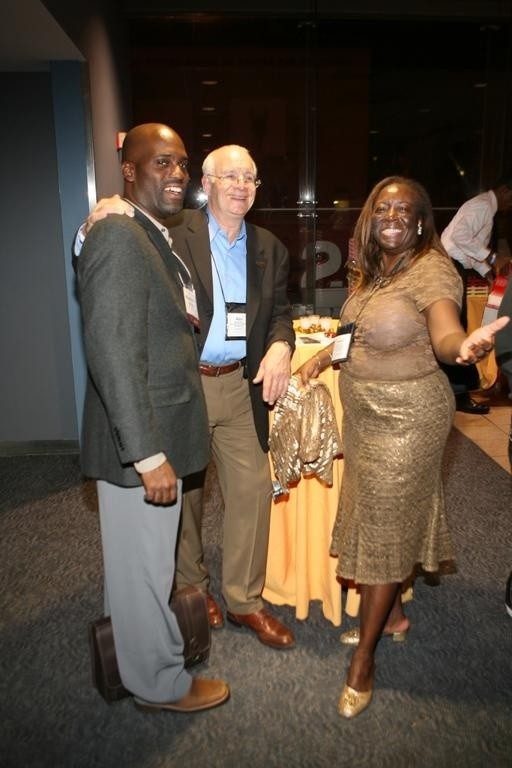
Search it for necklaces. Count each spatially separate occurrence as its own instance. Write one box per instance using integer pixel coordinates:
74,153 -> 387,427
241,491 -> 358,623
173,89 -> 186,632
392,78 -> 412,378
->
376,251 -> 404,287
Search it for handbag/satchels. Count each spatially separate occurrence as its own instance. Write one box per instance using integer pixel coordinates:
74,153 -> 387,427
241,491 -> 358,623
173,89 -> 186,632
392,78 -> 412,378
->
88,586 -> 211,706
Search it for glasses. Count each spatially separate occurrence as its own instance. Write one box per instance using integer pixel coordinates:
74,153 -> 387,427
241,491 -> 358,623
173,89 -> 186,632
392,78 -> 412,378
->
207,174 -> 262,188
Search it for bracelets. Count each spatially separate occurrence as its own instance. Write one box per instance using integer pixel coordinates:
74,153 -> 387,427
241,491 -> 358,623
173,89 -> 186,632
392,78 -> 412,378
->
324,347 -> 333,366
314,353 -> 322,374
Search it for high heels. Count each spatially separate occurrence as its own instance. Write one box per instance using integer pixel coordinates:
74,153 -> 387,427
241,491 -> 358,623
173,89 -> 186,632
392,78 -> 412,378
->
339,676 -> 372,718
340,626 -> 406,644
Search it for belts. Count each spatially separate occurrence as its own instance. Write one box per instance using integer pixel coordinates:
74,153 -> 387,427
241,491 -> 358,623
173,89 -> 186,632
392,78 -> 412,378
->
199,357 -> 246,378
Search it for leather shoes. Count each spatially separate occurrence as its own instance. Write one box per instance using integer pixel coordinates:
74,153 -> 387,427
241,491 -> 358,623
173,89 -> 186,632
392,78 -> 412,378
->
226,609 -> 295,649
206,590 -> 224,628
456,399 -> 489,414
133,679 -> 230,713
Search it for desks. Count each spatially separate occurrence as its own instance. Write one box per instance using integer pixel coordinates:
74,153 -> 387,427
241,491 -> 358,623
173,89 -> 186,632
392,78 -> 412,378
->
263,316 -> 360,624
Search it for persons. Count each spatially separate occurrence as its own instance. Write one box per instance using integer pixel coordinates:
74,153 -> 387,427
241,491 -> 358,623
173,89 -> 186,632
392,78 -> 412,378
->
73,143 -> 297,651
291,175 -> 512,720
439,179 -> 511,414
73,120 -> 231,714
